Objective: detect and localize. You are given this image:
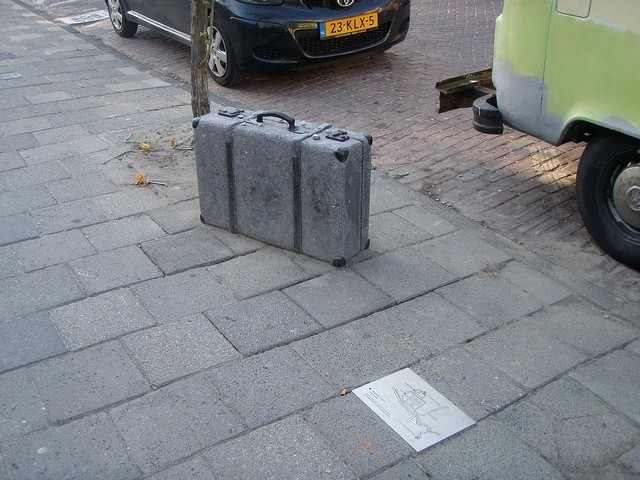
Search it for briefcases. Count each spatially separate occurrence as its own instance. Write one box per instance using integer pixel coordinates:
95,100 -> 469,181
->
191,107 -> 372,267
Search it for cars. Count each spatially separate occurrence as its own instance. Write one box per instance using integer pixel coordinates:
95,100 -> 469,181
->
106,0 -> 410,87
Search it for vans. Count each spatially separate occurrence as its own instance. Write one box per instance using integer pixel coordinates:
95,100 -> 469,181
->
437,2 -> 639,270
